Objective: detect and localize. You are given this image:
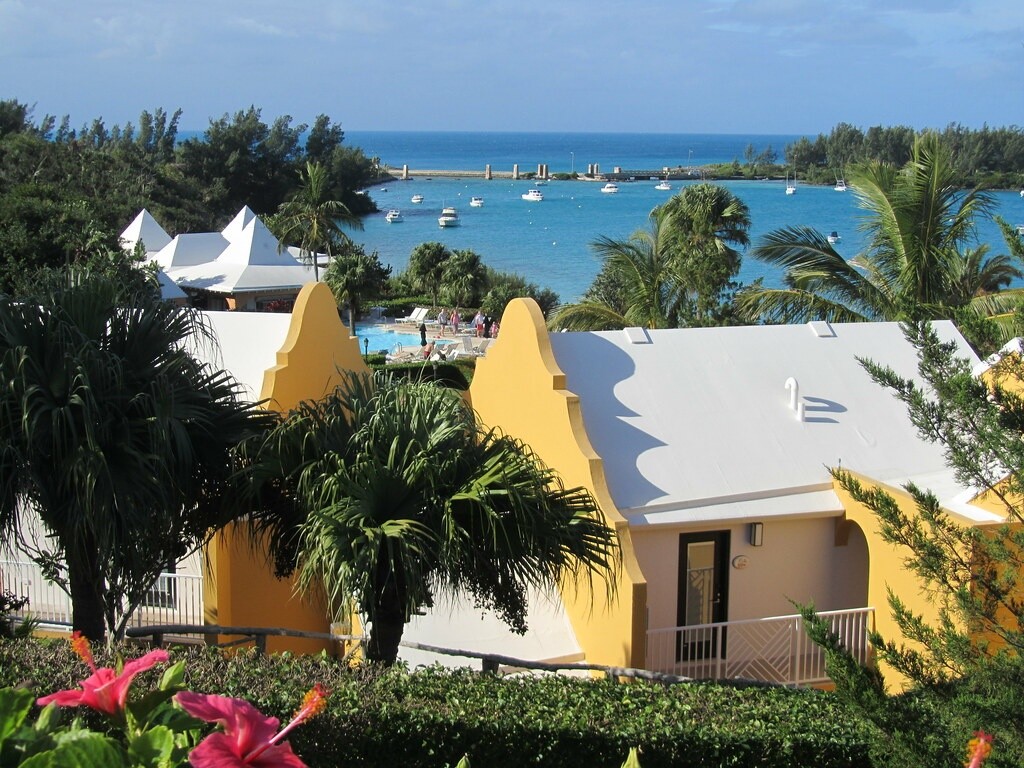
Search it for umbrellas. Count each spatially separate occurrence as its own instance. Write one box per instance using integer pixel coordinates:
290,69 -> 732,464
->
419,324 -> 427,346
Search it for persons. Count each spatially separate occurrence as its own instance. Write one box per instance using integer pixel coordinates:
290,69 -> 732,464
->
424,341 -> 435,355
475,311 -> 497,338
451,310 -> 460,335
291,293 -> 298,311
438,309 -> 447,336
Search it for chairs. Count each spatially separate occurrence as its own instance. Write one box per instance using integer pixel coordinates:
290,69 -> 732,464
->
385,307 -> 489,363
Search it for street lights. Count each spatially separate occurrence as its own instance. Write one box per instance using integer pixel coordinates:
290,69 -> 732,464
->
570,151 -> 574,173
793,155 -> 797,176
688,148 -> 693,168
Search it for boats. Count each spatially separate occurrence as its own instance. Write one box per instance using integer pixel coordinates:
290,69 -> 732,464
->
654,180 -> 672,190
521,190 -> 544,201
1019,190 -> 1024,197
385,209 -> 404,223
469,196 -> 484,208
600,183 -> 619,193
1016,225 -> 1024,236
827,231 -> 842,244
411,194 -> 424,203
437,200 -> 461,227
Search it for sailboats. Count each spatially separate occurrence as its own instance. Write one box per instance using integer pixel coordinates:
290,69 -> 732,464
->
785,171 -> 798,195
948,192 -> 975,228
833,170 -> 848,192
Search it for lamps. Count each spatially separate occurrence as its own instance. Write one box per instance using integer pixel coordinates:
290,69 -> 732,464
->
750,521 -> 763,546
